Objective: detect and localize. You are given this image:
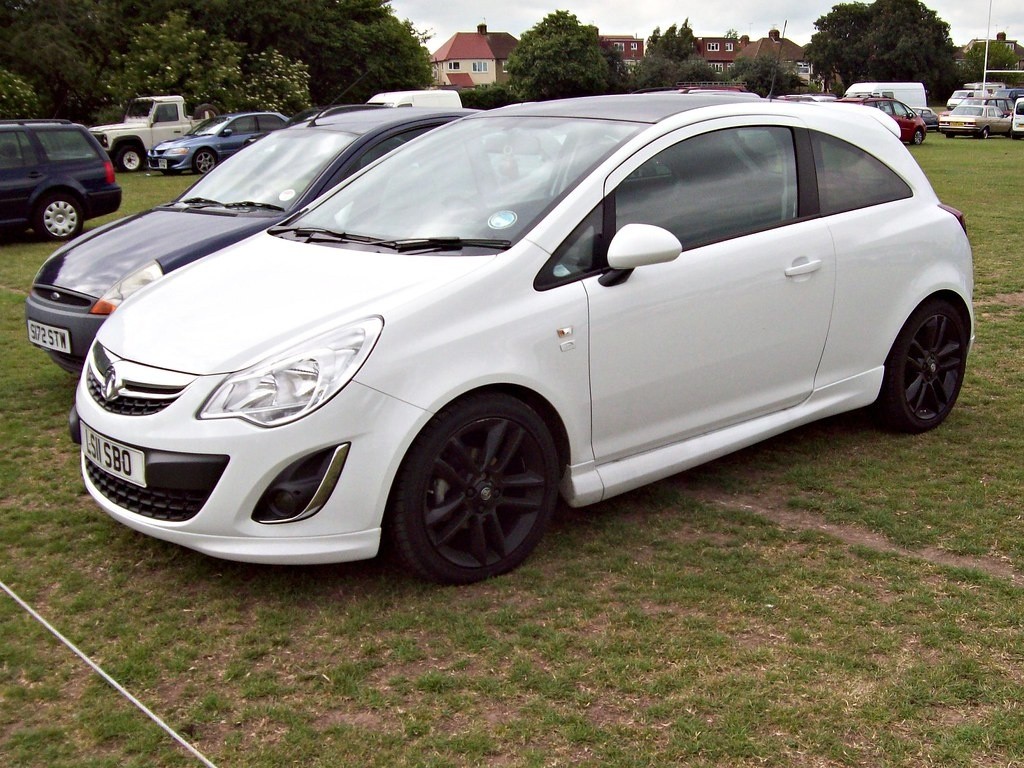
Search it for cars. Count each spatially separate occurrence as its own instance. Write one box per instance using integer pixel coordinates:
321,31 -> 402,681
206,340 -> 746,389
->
629,85 -> 841,106
22,103 -> 679,380
938,81 -> 1024,140
900,107 -> 941,131
0,116 -> 121,244
281,102 -> 387,128
145,111 -> 295,175
65,87 -> 976,585
830,92 -> 926,146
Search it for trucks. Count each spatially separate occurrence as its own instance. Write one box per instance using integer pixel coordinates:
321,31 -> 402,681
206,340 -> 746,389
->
86,94 -> 220,174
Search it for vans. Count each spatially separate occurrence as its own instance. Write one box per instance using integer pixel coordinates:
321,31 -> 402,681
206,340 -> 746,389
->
363,89 -> 465,113
844,82 -> 928,110
